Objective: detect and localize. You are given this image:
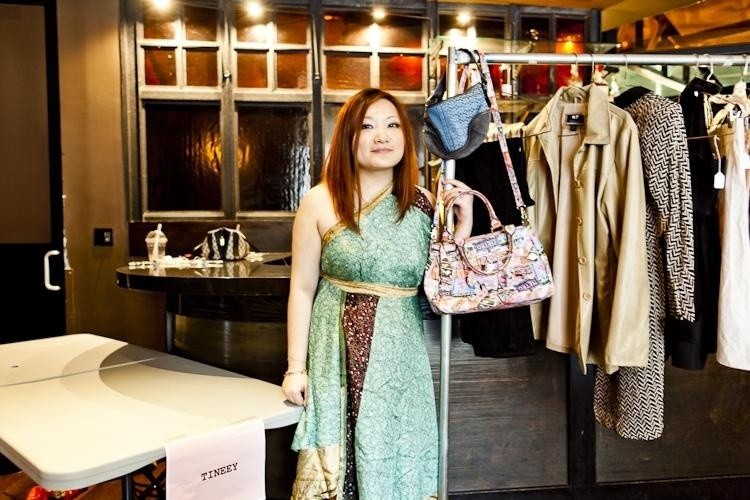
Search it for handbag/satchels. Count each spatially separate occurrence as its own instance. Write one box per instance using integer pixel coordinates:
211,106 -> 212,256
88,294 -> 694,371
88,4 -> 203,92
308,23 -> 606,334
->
420,189 -> 556,317
420,47 -> 492,160
192,223 -> 252,261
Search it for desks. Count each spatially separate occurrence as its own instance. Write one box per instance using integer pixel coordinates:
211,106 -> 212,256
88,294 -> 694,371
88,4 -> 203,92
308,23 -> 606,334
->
0,333 -> 304,500
115,252 -> 290,380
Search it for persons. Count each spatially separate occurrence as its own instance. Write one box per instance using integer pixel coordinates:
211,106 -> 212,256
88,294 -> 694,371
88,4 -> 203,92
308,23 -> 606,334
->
281,88 -> 474,500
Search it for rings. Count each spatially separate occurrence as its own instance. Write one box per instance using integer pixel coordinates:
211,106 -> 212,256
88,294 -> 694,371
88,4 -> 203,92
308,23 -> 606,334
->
458,191 -> 462,197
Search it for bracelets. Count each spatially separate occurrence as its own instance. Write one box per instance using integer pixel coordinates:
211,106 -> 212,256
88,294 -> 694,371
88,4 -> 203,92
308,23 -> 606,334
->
283,370 -> 307,375
286,357 -> 306,362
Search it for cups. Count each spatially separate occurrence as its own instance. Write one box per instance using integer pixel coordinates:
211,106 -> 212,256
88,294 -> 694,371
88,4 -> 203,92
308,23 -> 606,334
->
145,229 -> 168,269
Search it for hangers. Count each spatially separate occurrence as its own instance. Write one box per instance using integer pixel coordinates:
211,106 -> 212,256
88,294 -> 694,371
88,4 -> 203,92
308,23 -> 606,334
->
493,53 -> 750,140
